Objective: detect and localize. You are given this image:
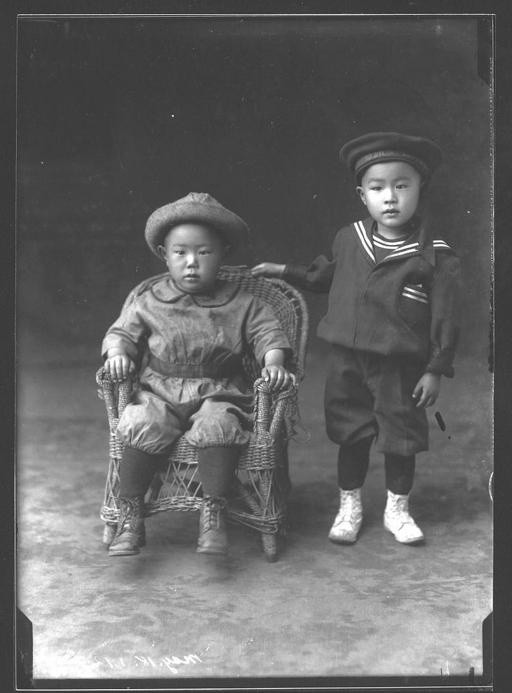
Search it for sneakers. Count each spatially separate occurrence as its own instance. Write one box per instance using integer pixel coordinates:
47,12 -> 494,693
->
380,489 -> 427,549
327,485 -> 367,550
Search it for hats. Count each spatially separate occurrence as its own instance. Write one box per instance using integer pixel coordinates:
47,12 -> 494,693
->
144,191 -> 251,263
335,130 -> 444,185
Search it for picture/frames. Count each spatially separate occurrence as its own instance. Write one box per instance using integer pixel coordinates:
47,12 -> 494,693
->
0,0 -> 511,692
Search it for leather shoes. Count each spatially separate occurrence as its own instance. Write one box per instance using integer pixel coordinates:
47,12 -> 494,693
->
106,494 -> 149,559
193,492 -> 232,561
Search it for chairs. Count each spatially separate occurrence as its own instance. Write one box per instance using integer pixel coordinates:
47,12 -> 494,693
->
94,266 -> 308,563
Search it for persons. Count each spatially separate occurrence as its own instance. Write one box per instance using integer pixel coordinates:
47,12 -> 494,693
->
100,192 -> 298,556
251,133 -> 464,544
